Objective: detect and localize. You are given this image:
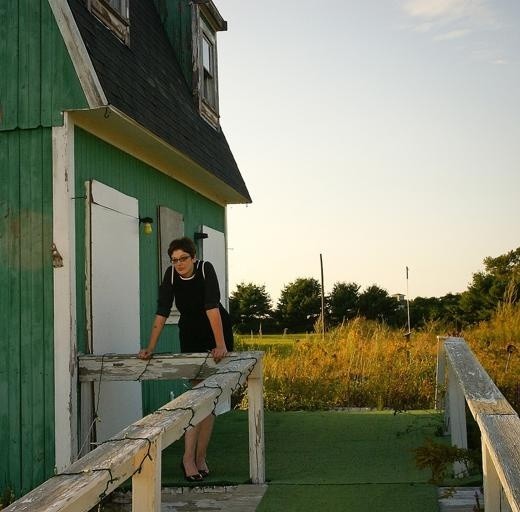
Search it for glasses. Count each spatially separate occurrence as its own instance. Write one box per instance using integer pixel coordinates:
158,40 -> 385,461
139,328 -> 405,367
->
170,256 -> 191,264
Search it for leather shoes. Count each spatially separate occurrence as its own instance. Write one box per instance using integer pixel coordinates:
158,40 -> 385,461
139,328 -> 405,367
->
195,457 -> 209,477
180,456 -> 203,481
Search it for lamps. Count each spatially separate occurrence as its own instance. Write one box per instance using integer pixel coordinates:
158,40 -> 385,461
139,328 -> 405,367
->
138,215 -> 153,234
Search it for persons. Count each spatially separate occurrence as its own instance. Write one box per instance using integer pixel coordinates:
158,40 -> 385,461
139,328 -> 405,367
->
136,237 -> 234,481
